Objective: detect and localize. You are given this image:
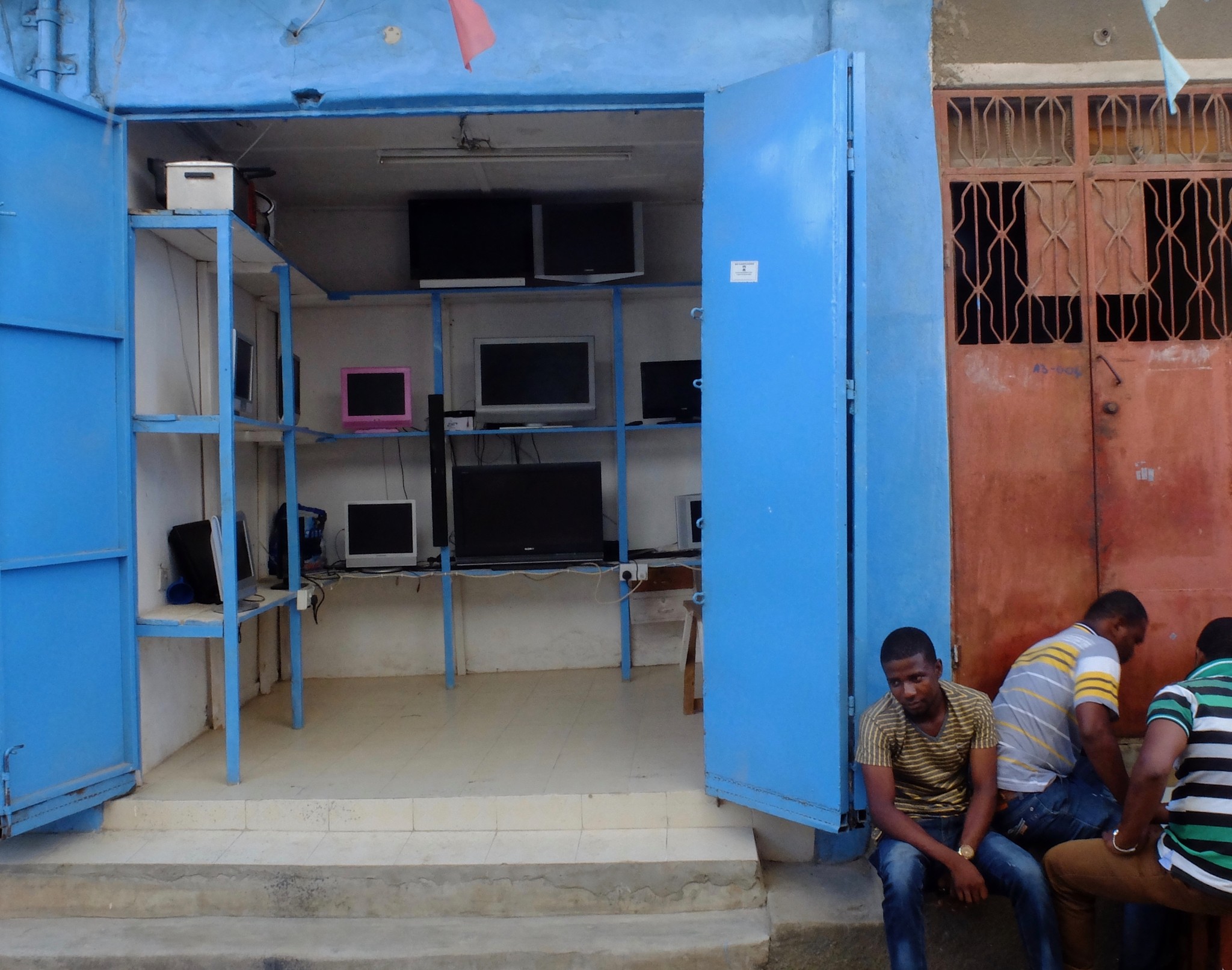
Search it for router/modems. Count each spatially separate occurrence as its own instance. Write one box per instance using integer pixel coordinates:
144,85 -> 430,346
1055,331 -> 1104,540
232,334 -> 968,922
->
443,410 -> 474,432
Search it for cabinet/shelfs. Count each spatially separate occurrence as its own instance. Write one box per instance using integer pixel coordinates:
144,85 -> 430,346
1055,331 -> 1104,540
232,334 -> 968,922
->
122,209 -> 327,790
333,278 -> 705,694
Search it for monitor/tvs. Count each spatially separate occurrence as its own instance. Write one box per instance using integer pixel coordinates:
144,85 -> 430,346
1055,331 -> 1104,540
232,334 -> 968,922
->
272,516 -> 308,590
407,198 -> 527,288
232,328 -> 255,415
339,367 -> 412,433
641,360 -> 700,424
675,493 -> 702,551
169,509 -> 259,612
451,463 -> 603,567
343,499 -> 417,573
279,354 -> 300,425
473,336 -> 597,429
533,196 -> 645,285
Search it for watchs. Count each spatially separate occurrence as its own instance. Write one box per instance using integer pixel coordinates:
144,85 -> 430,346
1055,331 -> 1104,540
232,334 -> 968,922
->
957,845 -> 974,860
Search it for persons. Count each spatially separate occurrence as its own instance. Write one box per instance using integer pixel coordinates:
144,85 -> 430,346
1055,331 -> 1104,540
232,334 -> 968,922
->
854,628 -> 1069,970
1043,618 -> 1232,970
993,589 -> 1162,969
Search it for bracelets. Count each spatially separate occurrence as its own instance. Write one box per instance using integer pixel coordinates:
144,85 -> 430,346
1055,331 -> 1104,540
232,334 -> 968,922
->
1113,829 -> 1138,852
